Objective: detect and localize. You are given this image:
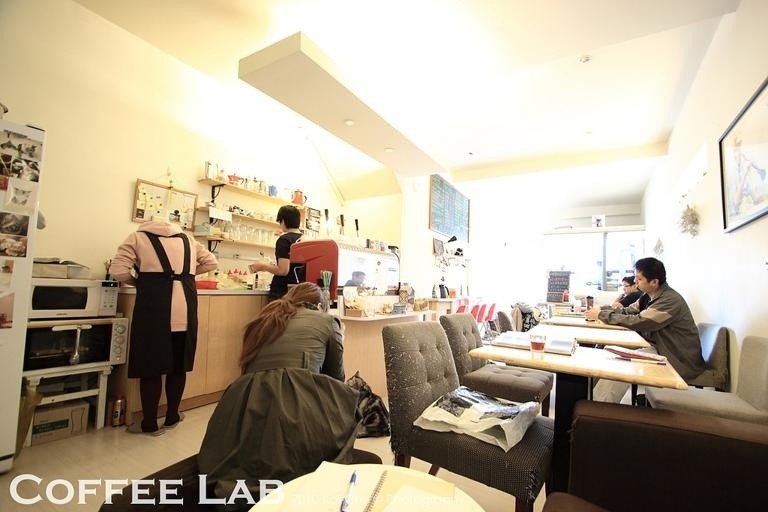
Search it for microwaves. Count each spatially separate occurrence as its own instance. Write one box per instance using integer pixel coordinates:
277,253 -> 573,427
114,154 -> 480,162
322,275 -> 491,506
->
25,277 -> 120,319
21,318 -> 128,376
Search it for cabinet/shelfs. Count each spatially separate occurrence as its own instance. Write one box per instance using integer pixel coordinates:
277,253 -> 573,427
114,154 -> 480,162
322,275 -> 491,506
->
195,175 -> 306,254
287,237 -> 402,302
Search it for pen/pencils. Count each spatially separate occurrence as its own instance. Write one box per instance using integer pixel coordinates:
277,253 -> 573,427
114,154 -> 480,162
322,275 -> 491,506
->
615,356 -> 666,365
342,469 -> 359,512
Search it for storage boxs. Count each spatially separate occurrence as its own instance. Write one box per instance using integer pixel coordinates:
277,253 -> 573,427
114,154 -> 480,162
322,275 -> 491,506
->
32,399 -> 90,446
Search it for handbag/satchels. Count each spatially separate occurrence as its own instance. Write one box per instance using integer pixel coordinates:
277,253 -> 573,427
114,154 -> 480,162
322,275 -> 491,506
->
414,385 -> 539,453
344,371 -> 390,438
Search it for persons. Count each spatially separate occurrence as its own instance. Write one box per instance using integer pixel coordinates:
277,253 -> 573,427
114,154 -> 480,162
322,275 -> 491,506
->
109,220 -> 217,437
344,271 -> 368,291
249,205 -> 307,302
613,277 -> 643,307
582,257 -> 705,404
199,282 -> 360,504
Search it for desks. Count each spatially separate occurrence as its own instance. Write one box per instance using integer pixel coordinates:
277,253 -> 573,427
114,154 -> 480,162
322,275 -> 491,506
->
23,365 -> 116,448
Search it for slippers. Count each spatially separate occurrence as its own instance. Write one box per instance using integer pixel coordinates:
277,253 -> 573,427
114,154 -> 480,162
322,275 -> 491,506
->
126,421 -> 165,436
160,412 -> 185,430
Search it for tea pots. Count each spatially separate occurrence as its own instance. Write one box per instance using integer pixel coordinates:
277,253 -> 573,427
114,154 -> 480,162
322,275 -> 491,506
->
228,172 -> 308,204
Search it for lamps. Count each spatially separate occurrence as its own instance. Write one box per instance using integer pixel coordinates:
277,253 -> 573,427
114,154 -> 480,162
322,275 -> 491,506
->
444,235 -> 457,245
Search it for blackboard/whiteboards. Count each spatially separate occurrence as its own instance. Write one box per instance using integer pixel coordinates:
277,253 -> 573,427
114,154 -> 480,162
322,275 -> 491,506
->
546,270 -> 570,303
428,173 -> 470,244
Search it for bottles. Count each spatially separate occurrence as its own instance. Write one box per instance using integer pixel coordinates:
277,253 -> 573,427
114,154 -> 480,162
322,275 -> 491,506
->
226,268 -> 248,282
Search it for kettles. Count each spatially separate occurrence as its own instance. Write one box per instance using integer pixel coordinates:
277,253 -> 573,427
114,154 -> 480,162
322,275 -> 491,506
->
438,284 -> 450,299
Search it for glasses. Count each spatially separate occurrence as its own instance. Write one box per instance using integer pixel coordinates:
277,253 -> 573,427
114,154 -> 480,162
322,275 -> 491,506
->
623,285 -> 632,288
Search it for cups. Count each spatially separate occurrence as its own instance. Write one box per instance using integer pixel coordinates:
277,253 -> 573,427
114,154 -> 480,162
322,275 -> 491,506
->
528,334 -> 546,353
222,218 -> 279,247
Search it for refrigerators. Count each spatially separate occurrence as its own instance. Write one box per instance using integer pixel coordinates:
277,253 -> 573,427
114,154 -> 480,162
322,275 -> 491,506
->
1,116 -> 47,477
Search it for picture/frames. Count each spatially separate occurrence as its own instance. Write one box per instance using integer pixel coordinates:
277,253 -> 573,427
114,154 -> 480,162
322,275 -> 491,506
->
717,74 -> 768,235
432,237 -> 445,254
